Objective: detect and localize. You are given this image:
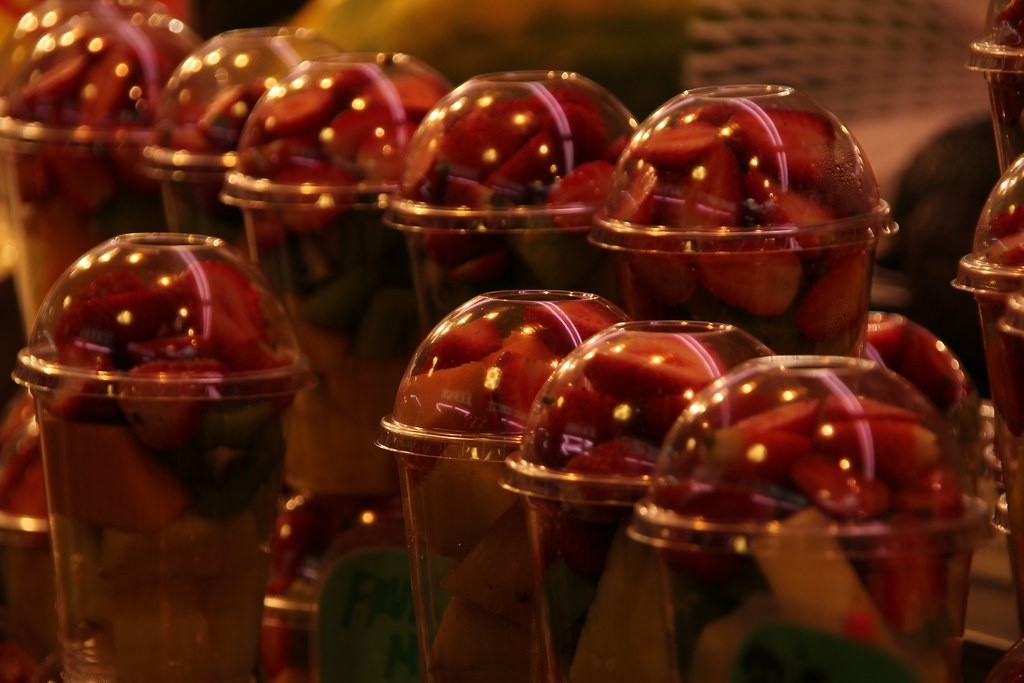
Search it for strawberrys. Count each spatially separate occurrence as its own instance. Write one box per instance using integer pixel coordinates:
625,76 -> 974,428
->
0,0 -> 1024,649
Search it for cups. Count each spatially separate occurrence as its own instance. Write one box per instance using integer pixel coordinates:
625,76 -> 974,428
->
0,0 -> 1024,683
